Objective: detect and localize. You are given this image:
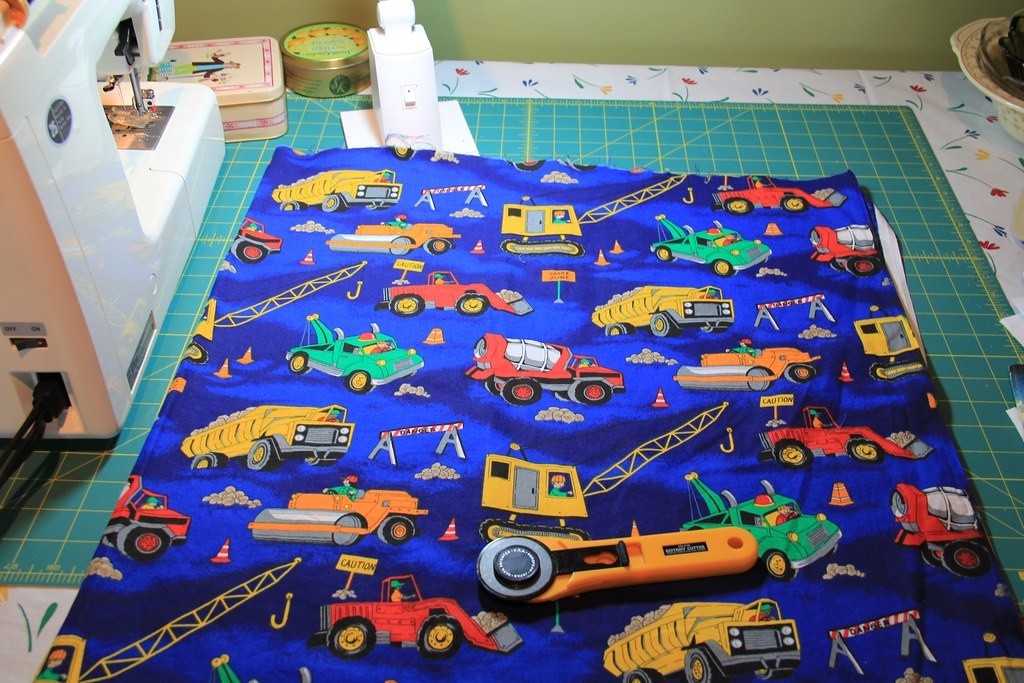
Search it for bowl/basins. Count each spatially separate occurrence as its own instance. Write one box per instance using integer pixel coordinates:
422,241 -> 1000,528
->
950,7 -> 1024,146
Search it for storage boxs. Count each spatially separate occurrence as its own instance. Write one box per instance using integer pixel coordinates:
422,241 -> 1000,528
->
119,36 -> 288,143
280,21 -> 371,97
